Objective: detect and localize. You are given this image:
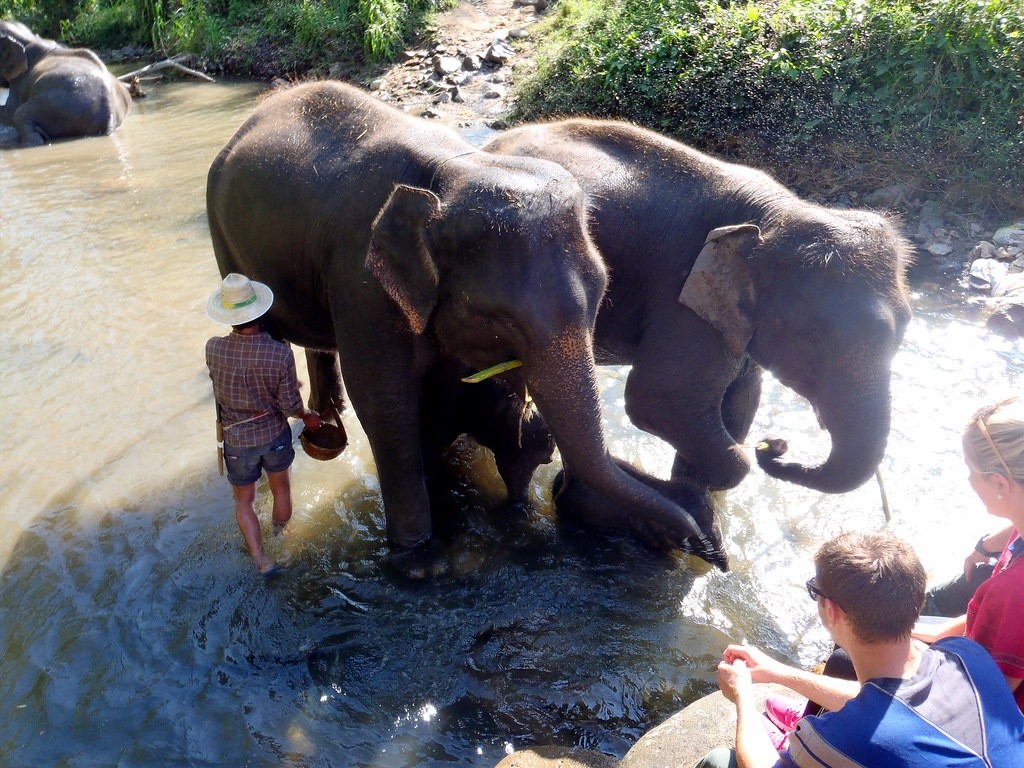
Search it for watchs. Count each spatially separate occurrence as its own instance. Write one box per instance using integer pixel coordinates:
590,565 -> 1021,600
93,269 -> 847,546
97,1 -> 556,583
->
975,532 -> 1003,560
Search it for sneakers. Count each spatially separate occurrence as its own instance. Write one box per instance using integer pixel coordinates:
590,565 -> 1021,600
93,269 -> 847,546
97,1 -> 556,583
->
768,730 -> 789,751
764,698 -> 802,733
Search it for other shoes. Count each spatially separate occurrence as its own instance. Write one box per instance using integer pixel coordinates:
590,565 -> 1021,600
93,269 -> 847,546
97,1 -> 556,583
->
262,567 -> 276,576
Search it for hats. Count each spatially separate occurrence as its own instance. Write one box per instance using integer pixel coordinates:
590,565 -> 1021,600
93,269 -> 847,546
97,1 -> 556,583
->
207,272 -> 274,326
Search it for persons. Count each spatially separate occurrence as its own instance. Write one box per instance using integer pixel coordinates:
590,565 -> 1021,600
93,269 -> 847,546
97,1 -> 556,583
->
762,397 -> 1024,754
206,272 -> 324,576
695,529 -> 1024,767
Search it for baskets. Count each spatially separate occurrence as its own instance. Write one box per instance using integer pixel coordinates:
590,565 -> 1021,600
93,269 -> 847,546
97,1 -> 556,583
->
297,396 -> 349,460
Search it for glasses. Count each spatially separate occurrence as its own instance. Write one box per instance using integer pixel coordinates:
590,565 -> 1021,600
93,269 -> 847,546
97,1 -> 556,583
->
806,578 -> 832,602
970,393 -> 1024,489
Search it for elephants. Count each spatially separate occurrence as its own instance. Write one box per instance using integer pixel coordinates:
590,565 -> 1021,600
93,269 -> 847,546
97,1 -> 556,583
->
0,18 -> 133,151
205,76 -> 916,575
